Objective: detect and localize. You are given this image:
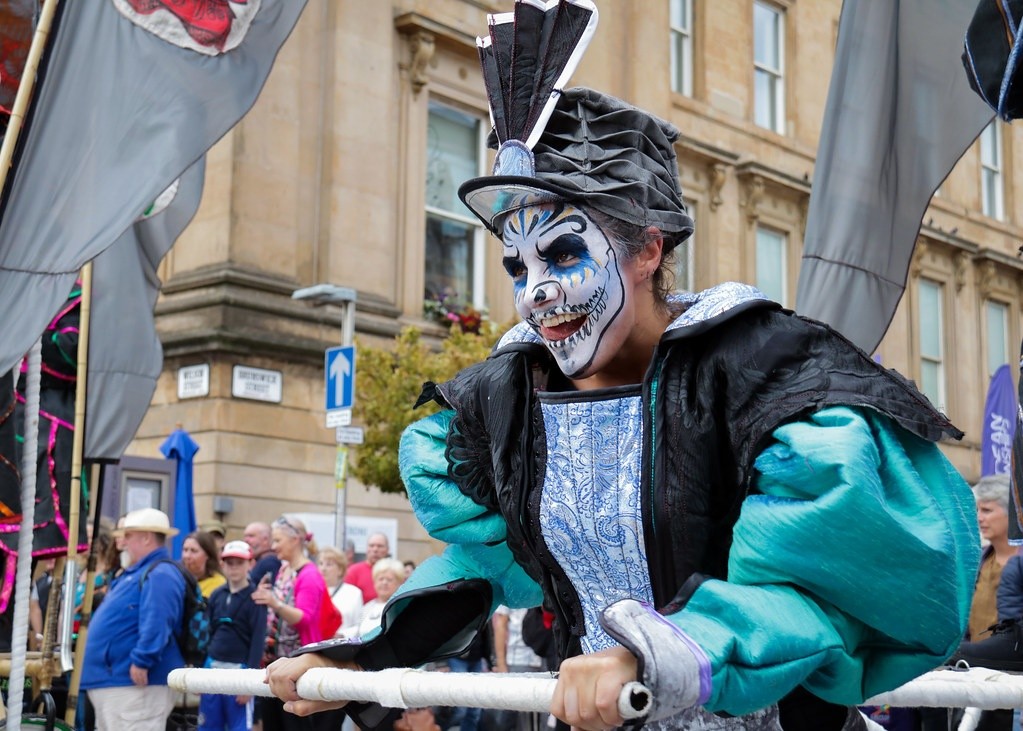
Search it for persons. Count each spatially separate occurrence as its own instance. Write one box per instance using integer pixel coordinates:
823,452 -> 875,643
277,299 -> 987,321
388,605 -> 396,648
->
29,513 -> 124,731
967,474 -> 1023,641
181,516 -> 415,731
263,86 -> 982,731
449,604 -> 547,731
80,508 -> 186,731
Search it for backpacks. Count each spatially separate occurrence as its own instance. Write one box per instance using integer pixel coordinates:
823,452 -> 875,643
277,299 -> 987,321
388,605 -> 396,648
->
140,559 -> 209,668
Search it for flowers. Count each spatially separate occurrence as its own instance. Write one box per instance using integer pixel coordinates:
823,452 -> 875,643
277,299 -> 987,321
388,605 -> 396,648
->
422,288 -> 487,331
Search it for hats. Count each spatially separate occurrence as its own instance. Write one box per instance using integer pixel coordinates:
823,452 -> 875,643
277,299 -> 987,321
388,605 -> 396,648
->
222,540 -> 253,560
111,507 -> 180,542
456,0 -> 694,254
200,520 -> 226,540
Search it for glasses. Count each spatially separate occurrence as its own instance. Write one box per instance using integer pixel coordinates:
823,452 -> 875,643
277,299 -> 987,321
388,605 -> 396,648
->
278,516 -> 299,533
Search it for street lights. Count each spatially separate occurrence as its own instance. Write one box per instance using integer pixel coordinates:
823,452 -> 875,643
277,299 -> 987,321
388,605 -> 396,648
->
291,282 -> 359,550
